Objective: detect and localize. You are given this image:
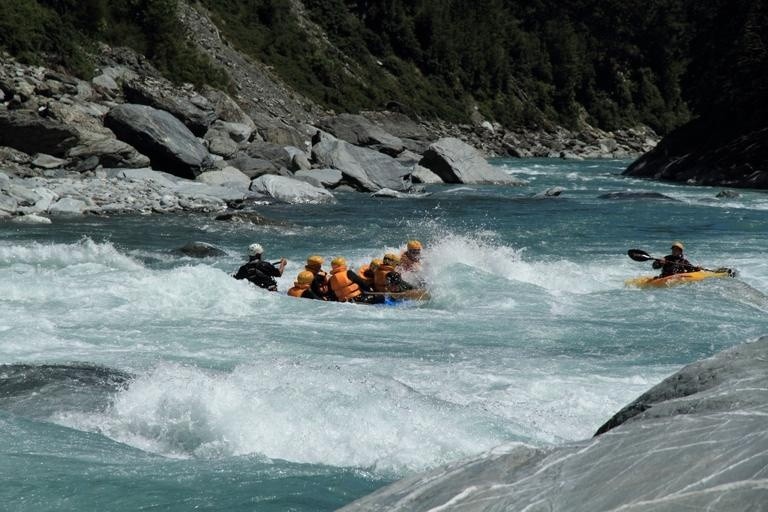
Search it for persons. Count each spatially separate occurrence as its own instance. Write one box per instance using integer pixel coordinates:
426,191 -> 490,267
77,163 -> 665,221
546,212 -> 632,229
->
652,241 -> 701,277
38,102 -> 57,119
312,130 -> 321,162
231,244 -> 287,294
288,240 -> 424,303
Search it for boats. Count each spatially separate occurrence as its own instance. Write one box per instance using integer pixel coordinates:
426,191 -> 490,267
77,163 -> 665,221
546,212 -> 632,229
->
356,287 -> 431,310
622,265 -> 740,290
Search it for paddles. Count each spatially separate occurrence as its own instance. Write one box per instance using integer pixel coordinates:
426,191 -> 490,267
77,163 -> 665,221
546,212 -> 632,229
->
628,249 -> 736,277
362,289 -> 431,301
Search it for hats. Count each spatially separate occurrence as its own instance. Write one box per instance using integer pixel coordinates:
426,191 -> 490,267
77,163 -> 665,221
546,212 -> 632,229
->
331,257 -> 347,270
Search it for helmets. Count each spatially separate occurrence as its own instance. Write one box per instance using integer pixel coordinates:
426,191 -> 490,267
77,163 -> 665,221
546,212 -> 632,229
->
370,240 -> 422,273
297,255 -> 324,285
671,240 -> 685,250
247,243 -> 265,257
